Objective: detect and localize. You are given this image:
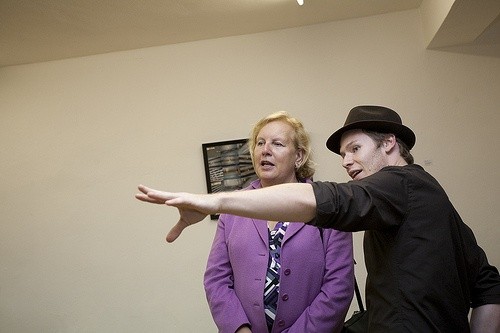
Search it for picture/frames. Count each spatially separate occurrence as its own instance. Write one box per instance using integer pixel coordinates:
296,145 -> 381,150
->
202,139 -> 259,221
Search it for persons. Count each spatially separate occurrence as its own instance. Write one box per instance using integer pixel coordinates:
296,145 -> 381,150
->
135,105 -> 500,333
203,111 -> 356,333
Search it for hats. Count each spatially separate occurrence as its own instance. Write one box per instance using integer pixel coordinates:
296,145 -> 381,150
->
327,105 -> 416,155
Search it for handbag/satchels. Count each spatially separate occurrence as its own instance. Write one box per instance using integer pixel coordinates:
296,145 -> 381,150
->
343,310 -> 369,333
